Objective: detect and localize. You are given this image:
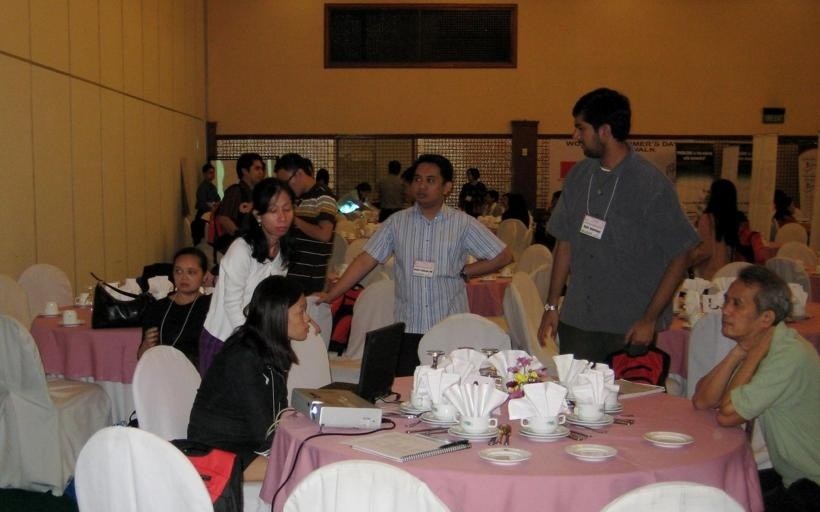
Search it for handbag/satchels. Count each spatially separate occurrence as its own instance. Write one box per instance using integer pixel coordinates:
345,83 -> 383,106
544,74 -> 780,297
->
170,439 -> 241,512
92,281 -> 155,329
737,219 -> 767,265
609,346 -> 670,387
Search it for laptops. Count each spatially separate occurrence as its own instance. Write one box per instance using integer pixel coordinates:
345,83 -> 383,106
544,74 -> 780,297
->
319,321 -> 406,403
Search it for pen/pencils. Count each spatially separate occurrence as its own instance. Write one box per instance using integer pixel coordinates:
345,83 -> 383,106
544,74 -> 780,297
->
439,440 -> 468,449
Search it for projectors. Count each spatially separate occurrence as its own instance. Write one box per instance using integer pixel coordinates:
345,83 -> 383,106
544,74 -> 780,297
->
291,388 -> 383,430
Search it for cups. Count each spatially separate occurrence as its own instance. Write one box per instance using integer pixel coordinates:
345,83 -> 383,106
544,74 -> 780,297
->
44,293 -> 90,323
498,267 -> 514,274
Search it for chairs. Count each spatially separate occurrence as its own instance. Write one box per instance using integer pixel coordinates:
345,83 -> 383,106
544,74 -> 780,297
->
0,272 -> 31,332
73,425 -> 215,512
132,344 -> 203,443
601,482 -> 745,512
0,315 -> 112,497
17,262 -> 74,319
773,223 -> 809,248
283,458 -> 448,511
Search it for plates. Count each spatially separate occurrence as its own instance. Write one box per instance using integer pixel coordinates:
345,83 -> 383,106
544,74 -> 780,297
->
57,320 -> 85,328
479,278 -> 496,281
40,312 -> 63,317
641,431 -> 694,448
497,274 -> 513,278
399,403 -> 623,465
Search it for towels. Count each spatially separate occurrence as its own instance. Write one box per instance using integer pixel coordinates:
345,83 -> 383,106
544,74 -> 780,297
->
413,350 -> 620,418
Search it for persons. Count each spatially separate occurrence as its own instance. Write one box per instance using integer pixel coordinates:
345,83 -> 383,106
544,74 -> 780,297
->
685,179 -> 806,281
690,265 -> 820,512
137,152 -> 561,483
538,87 -> 706,369
